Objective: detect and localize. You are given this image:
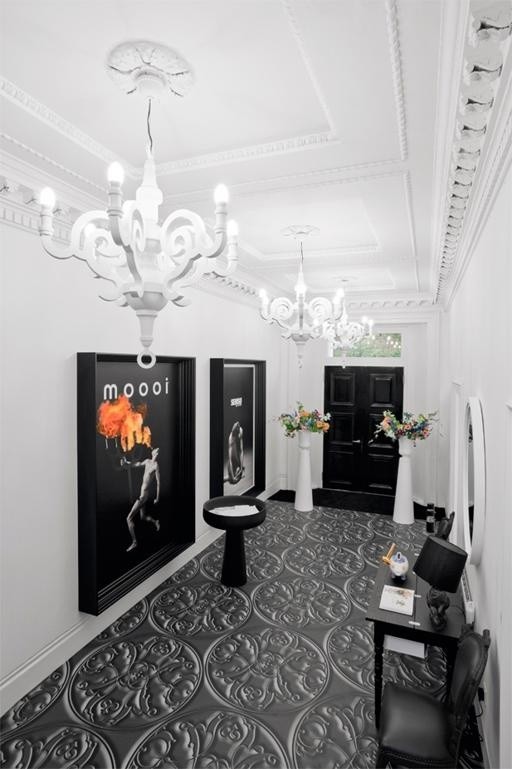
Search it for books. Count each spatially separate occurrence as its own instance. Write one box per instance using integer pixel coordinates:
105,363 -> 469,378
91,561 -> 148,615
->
378,584 -> 415,616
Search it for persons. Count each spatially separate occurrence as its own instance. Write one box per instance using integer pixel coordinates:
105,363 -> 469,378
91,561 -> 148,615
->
121,448 -> 160,552
228,421 -> 245,484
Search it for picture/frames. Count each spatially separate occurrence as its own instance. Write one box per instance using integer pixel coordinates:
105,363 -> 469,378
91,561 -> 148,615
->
210,357 -> 266,504
75,351 -> 196,617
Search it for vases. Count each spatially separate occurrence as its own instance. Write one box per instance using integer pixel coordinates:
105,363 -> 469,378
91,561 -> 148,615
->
398,435 -> 415,457
299,430 -> 310,449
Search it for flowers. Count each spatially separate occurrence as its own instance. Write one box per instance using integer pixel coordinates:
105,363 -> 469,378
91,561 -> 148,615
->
278,401 -> 331,439
374,410 -> 439,448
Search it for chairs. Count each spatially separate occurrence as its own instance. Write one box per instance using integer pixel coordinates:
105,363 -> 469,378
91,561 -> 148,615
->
435,511 -> 455,540
376,629 -> 491,769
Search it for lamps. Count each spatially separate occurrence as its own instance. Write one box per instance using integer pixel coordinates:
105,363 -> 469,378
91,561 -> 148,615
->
413,537 -> 466,630
259,232 -> 344,367
325,280 -> 373,369
37,74 -> 240,368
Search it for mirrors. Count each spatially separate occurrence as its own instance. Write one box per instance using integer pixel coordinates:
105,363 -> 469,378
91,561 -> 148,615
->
461,398 -> 486,565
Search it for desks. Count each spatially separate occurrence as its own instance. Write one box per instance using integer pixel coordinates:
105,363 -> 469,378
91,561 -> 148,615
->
365,546 -> 466,732
